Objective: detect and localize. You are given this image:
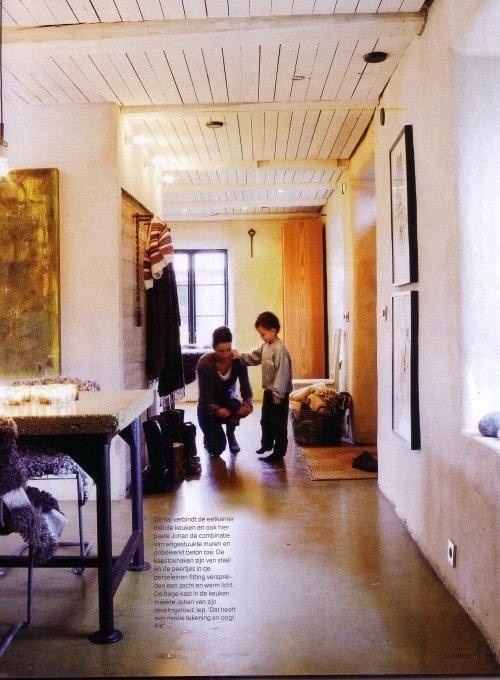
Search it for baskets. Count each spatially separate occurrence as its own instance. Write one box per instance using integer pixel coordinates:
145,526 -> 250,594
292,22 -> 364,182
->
289,406 -> 344,445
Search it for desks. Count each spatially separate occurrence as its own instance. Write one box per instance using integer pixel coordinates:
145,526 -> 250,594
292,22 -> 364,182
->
0,388 -> 155,644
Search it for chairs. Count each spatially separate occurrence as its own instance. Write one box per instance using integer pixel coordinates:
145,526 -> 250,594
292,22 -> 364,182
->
0,415 -> 66,658
0,377 -> 101,578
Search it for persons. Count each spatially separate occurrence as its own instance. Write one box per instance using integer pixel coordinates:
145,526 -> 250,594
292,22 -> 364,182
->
231,311 -> 293,465
196,326 -> 253,456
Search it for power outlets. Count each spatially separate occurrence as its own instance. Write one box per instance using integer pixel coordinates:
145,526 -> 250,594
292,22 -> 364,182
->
447,540 -> 456,567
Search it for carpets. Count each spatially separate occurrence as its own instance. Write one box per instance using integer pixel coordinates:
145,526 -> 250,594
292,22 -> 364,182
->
299,444 -> 378,481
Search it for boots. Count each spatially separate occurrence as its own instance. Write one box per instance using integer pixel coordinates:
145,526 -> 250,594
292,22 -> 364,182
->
143,409 -> 201,495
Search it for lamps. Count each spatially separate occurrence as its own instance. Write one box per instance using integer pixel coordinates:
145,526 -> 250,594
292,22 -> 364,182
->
0,0 -> 9,177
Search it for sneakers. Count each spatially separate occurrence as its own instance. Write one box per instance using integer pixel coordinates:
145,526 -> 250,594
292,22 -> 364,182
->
352,451 -> 378,472
